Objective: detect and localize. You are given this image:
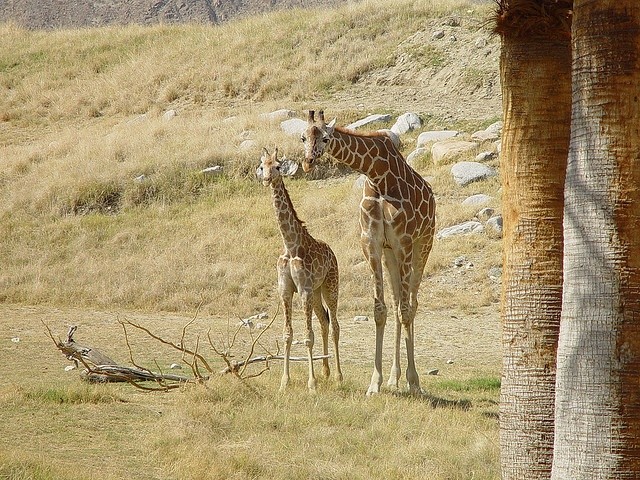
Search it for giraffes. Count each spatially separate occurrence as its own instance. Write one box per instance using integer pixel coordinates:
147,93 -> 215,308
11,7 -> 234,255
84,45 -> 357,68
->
258,146 -> 344,395
299,107 -> 436,398
